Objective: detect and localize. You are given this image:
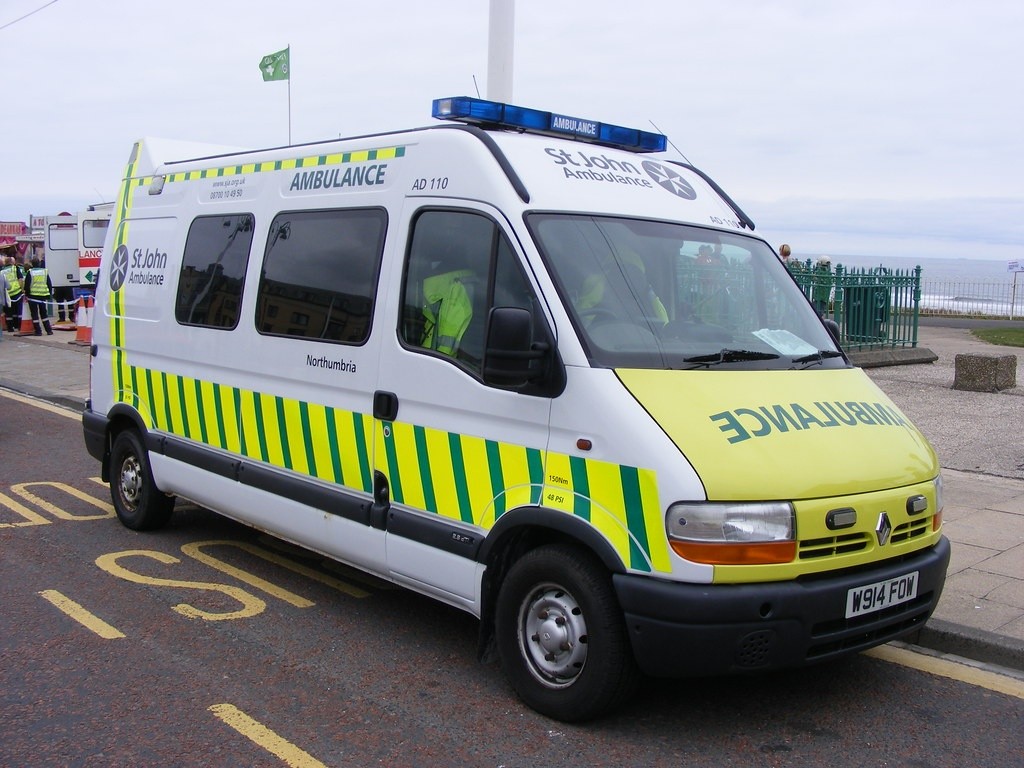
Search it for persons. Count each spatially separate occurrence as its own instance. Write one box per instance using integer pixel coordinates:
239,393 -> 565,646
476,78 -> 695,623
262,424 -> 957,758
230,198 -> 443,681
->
690,243 -> 731,313
779,244 -> 806,273
53,286 -> 76,323
24,258 -> 52,336
0,255 -> 23,337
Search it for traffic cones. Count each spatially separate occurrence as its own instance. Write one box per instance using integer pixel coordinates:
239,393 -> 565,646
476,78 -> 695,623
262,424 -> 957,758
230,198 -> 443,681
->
68,295 -> 86,344
13,295 -> 37,337
75,295 -> 94,346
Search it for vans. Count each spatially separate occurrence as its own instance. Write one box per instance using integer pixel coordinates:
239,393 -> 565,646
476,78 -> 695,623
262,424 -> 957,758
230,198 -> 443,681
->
45,204 -> 115,287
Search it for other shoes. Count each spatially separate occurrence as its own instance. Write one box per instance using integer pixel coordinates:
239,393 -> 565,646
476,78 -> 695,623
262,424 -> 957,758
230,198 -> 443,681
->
47,331 -> 53,335
34,331 -> 42,336
8,327 -> 14,332
57,318 -> 65,321
70,317 -> 74,322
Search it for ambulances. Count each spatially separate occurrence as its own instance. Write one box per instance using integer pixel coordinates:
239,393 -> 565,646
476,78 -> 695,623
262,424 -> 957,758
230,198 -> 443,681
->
82,91 -> 948,720
